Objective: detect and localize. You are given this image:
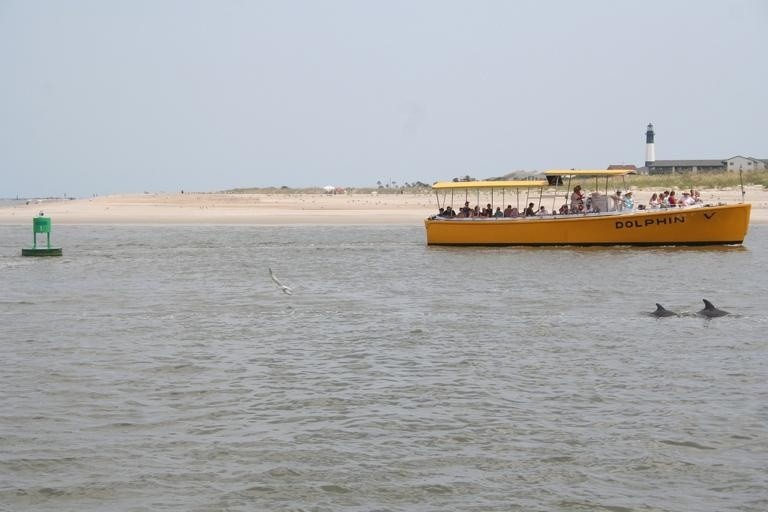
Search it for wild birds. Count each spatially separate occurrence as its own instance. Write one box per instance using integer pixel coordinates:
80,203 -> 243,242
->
269,267 -> 293,296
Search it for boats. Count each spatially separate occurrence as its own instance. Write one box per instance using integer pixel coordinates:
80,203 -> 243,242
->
423,169 -> 753,247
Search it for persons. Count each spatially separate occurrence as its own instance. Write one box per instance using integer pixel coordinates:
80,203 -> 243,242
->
434,183 -> 704,218
